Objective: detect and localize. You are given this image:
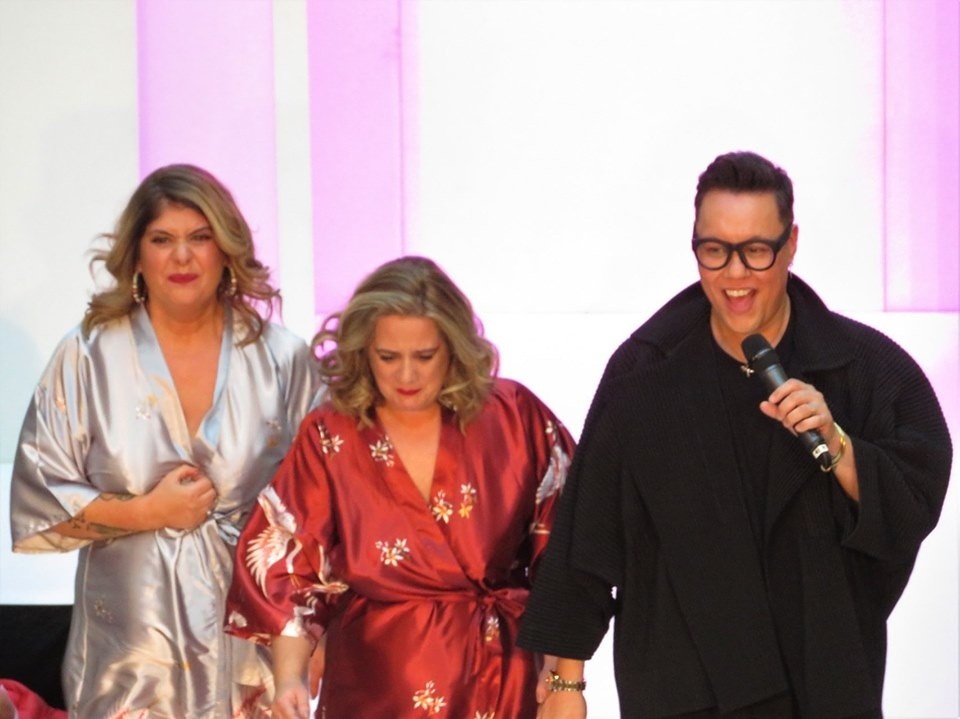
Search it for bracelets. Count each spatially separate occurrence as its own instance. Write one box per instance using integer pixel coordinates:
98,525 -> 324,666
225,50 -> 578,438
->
821,421 -> 847,473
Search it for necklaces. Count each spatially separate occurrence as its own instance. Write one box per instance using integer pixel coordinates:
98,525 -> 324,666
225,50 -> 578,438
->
710,298 -> 787,379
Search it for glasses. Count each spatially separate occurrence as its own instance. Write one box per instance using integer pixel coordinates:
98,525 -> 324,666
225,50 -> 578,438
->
691,217 -> 794,272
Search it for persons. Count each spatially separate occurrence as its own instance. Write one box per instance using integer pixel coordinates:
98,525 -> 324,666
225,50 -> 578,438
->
222,257 -> 579,719
10,163 -> 336,719
516,151 -> 955,719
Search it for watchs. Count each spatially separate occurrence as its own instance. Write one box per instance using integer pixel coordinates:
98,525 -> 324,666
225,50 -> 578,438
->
544,671 -> 586,694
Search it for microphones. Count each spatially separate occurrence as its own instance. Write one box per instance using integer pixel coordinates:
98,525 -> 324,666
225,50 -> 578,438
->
739,332 -> 837,474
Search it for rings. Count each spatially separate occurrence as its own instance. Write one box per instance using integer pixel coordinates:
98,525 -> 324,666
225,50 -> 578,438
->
206,509 -> 212,519
807,402 -> 820,417
